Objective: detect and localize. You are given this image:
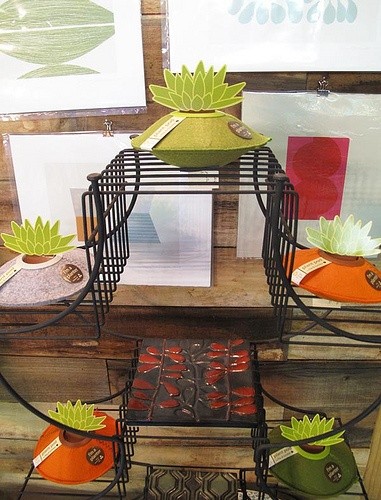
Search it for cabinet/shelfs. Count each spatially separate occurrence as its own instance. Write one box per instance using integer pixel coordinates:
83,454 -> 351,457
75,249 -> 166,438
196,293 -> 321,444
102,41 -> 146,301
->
0,134 -> 381,500
